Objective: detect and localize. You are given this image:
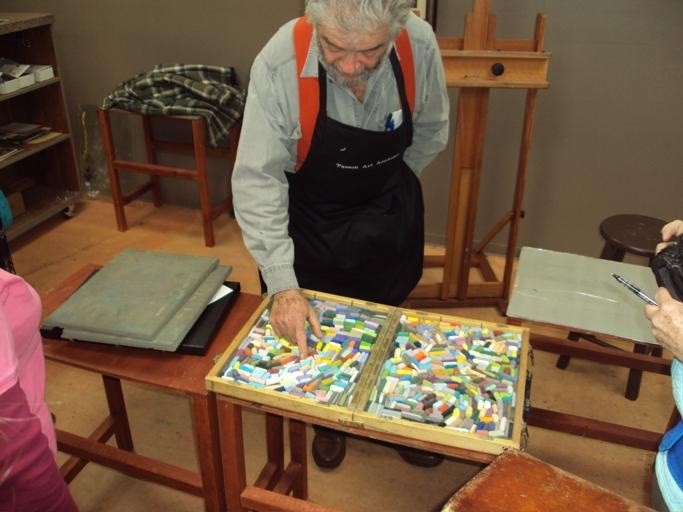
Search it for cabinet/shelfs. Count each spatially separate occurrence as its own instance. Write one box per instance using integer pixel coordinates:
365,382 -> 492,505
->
0,12 -> 84,245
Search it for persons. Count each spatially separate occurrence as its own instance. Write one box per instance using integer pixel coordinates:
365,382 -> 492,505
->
231,0 -> 458,468
646,218 -> 683,420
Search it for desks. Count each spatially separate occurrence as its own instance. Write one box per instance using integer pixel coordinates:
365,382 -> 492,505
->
509,246 -> 661,391
443,448 -> 654,511
207,284 -> 532,510
96,105 -> 240,250
34,263 -> 264,510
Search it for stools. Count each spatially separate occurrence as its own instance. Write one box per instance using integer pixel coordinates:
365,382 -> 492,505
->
558,213 -> 668,401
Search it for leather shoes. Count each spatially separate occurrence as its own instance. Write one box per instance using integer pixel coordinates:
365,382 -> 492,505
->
312,430 -> 345,467
394,444 -> 445,467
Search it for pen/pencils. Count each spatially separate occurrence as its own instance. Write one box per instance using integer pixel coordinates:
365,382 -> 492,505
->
384,111 -> 395,130
609,270 -> 658,307
